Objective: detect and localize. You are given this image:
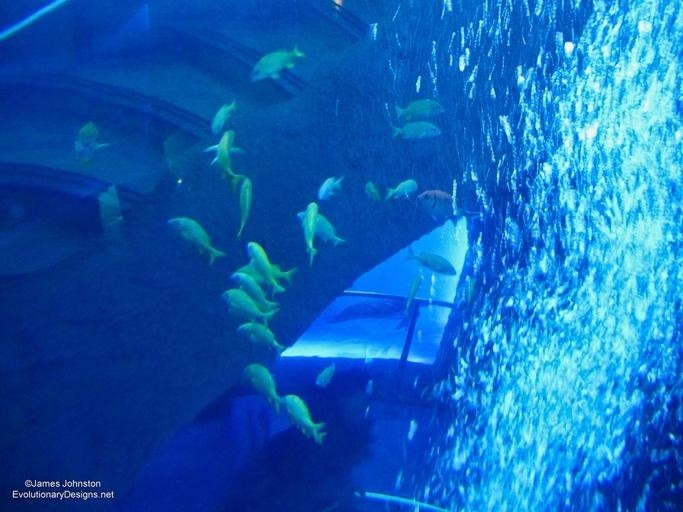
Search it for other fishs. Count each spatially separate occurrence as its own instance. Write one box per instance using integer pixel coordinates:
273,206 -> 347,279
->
388,97 -> 450,142
167,96 -> 345,349
